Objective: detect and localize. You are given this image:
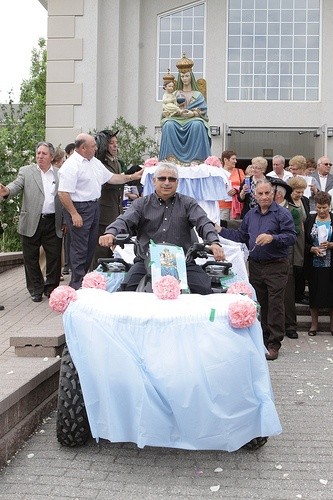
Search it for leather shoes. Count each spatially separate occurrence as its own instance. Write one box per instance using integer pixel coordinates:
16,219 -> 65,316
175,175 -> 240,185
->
286,330 -> 298,338
44,292 -> 50,298
32,294 -> 42,301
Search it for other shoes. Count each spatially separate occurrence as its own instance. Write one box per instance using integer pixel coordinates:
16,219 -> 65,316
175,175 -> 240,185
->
266,349 -> 278,360
60,274 -> 64,280
308,327 -> 320,336
297,298 -> 310,304
63,264 -> 70,274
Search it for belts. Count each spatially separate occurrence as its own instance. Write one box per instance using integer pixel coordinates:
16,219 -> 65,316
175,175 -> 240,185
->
41,214 -> 55,218
72,200 -> 97,203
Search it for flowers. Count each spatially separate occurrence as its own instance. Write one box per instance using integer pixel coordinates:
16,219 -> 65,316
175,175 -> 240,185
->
155,275 -> 181,299
228,301 -> 257,329
228,283 -> 253,299
81,271 -> 106,292
206,156 -> 221,167
48,286 -> 76,312
144,156 -> 158,168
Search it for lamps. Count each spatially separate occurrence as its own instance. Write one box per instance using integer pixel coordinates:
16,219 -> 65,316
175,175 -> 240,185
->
327,130 -> 332,137
210,125 -> 220,136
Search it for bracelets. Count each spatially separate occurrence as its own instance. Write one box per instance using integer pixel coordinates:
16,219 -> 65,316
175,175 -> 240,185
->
130,175 -> 132,181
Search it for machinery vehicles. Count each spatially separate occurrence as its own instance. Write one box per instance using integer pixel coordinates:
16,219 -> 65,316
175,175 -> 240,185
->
57,234 -> 270,451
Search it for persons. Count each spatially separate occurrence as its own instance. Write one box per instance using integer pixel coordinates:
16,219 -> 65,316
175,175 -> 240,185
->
214,181 -> 297,361
218,150 -> 245,221
57,132 -> 145,291
161,80 -> 186,118
88,130 -> 129,272
97,164 -> 226,295
122,165 -> 144,213
158,69 -> 212,162
0,142 -> 67,301
237,154 -> 333,339
38,143 -> 78,281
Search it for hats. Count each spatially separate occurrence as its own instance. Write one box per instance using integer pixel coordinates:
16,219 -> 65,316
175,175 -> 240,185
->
270,179 -> 292,202
102,130 -> 120,136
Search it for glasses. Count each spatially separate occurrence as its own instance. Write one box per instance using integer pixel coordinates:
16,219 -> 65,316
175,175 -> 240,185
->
156,176 -> 177,182
320,163 -> 332,166
